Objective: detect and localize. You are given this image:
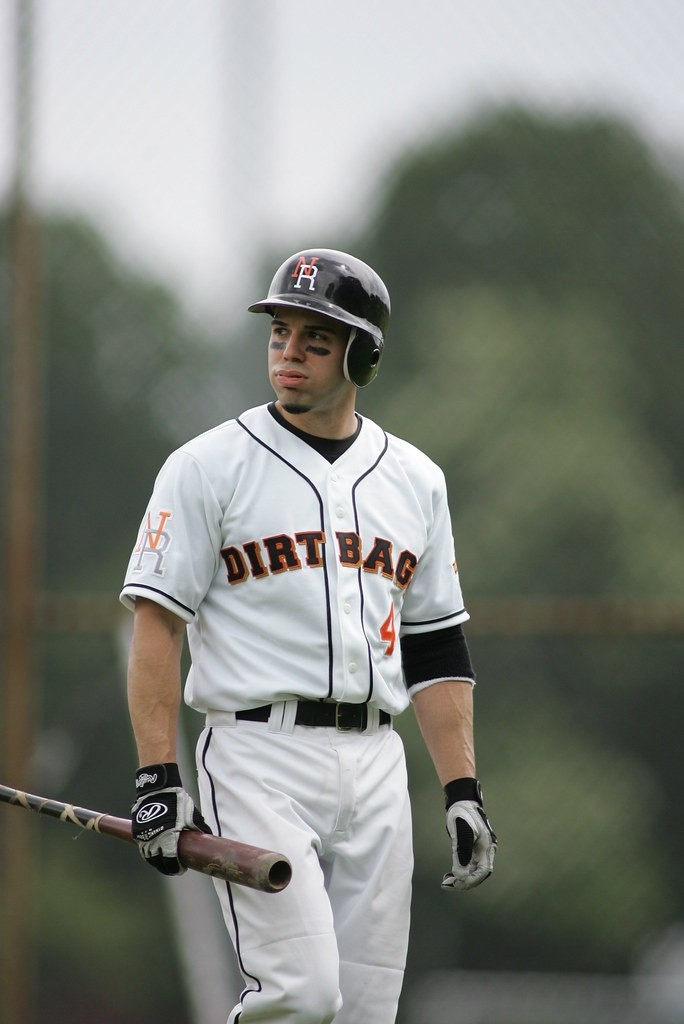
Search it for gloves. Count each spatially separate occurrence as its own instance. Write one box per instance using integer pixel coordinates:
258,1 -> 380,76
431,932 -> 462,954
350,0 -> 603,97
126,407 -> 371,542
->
440,777 -> 498,891
131,762 -> 213,876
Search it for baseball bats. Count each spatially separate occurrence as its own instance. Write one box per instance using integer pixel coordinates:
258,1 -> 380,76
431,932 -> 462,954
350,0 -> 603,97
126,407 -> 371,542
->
0,783 -> 293,895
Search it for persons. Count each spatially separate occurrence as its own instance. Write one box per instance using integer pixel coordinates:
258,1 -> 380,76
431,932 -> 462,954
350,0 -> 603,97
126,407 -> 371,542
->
117,244 -> 497,1020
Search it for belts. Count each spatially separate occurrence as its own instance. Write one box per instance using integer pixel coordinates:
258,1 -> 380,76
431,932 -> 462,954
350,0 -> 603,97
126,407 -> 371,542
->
236,700 -> 391,733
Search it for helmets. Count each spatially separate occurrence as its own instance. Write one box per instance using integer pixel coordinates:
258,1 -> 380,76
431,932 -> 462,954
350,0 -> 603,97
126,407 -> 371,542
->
248,248 -> 392,388
